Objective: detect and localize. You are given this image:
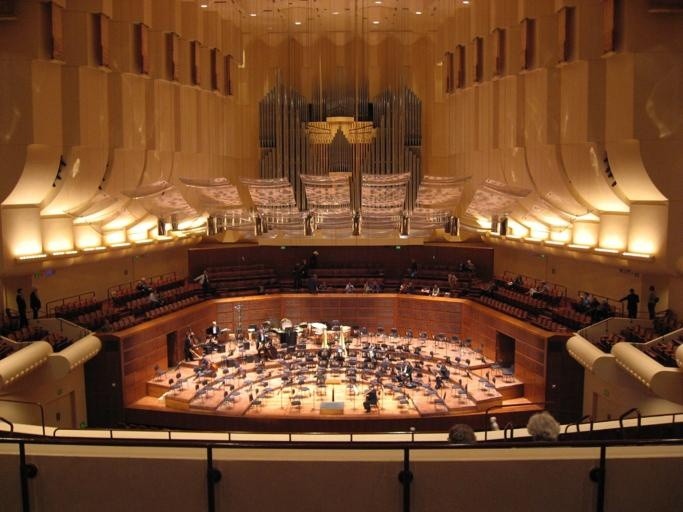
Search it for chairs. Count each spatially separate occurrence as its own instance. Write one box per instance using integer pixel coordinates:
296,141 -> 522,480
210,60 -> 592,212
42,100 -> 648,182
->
152,319 -> 517,415
480,272 -> 622,334
46,263 -> 281,331
299,258 -> 472,297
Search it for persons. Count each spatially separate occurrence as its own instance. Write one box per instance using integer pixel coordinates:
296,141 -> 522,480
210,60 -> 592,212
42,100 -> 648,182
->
16,287 -> 32,335
30,287 -> 41,320
136,278 -> 160,309
295,260 -> 319,294
344,260 -> 476,297
485,274 -> 676,336
449,424 -> 476,444
527,413 -> 560,441
184,320 -> 449,412
194,270 -> 210,298
490,416 -> 499,430
